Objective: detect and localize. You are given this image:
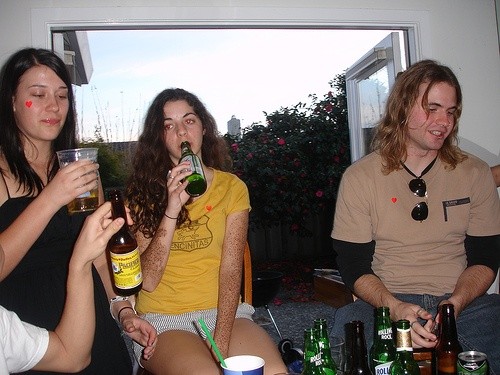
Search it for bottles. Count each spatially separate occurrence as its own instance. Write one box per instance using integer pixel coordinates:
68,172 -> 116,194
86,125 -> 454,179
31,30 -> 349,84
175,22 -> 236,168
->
178,141 -> 208,197
104,189 -> 143,297
301,305 -> 422,375
430,303 -> 463,375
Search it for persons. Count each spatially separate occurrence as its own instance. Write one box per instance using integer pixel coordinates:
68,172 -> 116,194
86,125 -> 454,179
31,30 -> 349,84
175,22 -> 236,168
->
330,59 -> 500,375
0,202 -> 134,375
0,47 -> 158,375
128,88 -> 288,375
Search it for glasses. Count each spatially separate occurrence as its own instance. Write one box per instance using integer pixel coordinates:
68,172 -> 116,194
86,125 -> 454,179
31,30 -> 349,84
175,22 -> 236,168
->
409,177 -> 429,222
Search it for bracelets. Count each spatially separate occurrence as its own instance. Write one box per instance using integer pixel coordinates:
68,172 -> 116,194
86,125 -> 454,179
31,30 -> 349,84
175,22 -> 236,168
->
109,295 -> 128,319
118,307 -> 134,321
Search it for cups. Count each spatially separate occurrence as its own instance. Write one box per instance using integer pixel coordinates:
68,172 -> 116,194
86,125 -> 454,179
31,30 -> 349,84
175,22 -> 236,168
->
56,148 -> 100,213
328,334 -> 346,375
220,355 -> 266,375
